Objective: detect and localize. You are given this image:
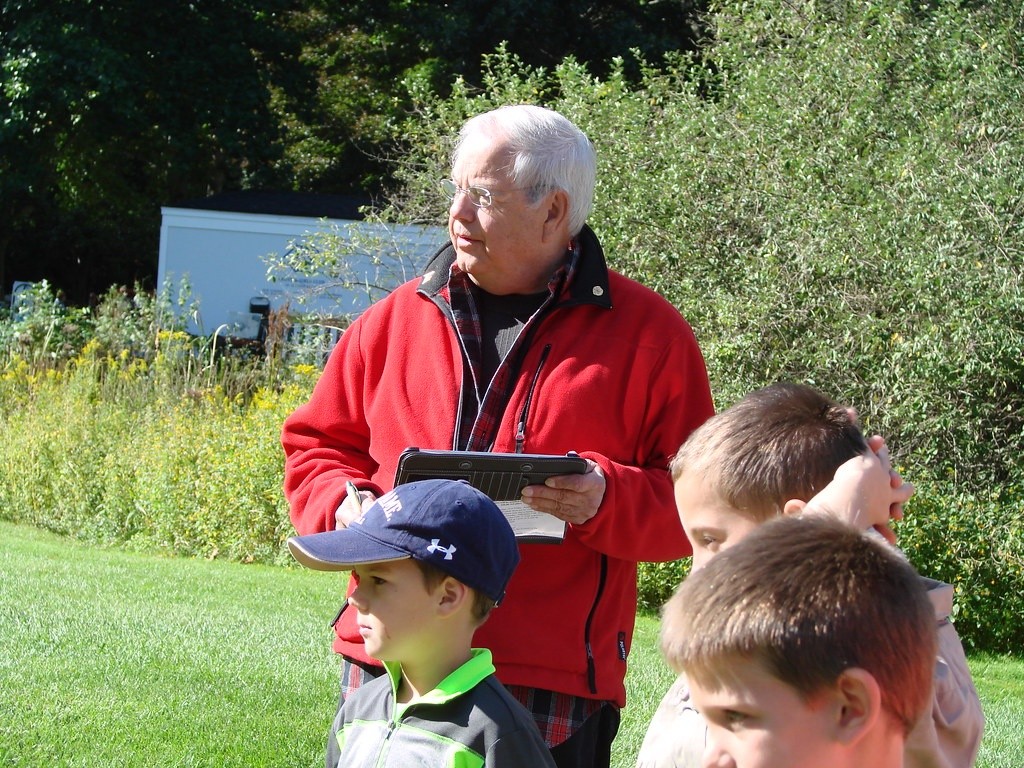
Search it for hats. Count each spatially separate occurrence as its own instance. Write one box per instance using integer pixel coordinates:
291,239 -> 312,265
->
287,475 -> 522,609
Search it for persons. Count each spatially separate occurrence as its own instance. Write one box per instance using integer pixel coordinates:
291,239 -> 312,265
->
635,383 -> 985,766
281,106 -> 719,768
658,513 -> 939,768
320,479 -> 558,768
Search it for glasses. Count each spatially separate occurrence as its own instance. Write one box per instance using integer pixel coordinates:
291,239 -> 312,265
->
439,176 -> 543,209
